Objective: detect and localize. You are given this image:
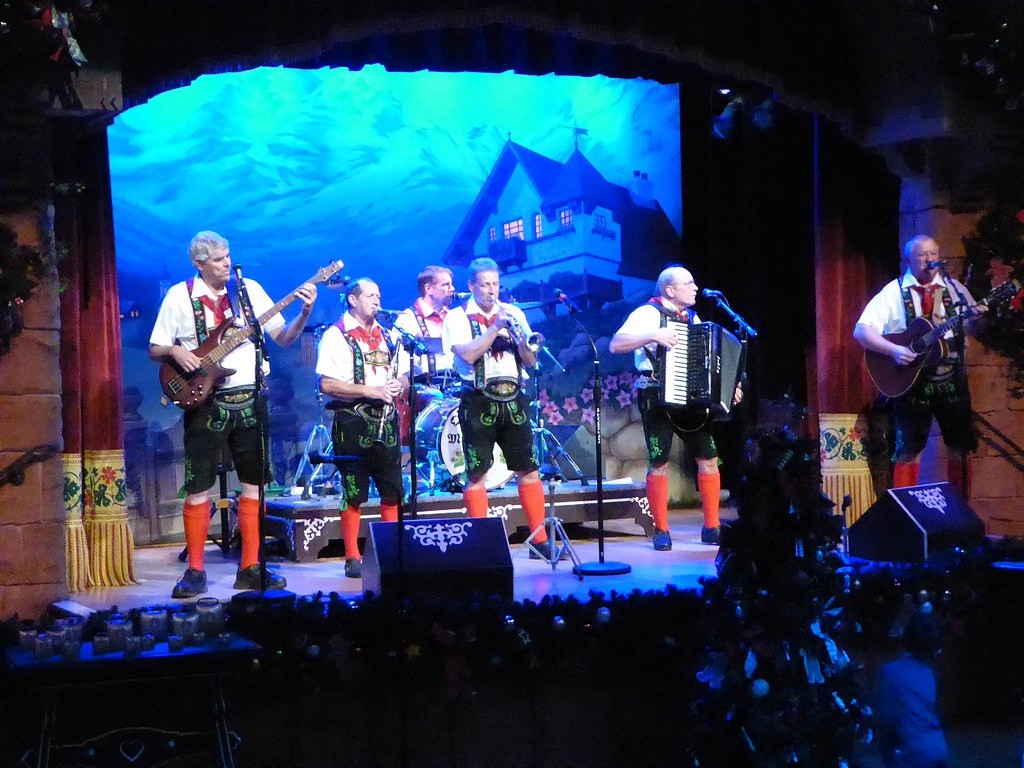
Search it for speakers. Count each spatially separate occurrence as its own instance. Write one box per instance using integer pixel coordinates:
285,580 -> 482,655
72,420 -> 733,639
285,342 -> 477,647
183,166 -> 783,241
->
849,482 -> 984,558
363,516 -> 513,608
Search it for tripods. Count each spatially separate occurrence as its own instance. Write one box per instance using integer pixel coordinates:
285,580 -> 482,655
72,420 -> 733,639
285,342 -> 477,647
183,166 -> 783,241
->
513,472 -> 585,583
286,316 -> 434,499
177,452 -> 239,562
530,353 -> 588,489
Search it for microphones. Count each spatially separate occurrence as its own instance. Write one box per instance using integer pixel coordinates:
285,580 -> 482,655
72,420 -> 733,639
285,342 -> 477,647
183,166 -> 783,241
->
232,264 -> 243,279
927,259 -> 946,269
452,291 -> 474,300
373,306 -> 403,314
701,288 -> 723,297
554,287 -> 580,312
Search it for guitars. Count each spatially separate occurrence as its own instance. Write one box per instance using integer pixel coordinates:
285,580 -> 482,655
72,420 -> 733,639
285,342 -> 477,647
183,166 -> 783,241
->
157,259 -> 344,410
864,278 -> 1023,398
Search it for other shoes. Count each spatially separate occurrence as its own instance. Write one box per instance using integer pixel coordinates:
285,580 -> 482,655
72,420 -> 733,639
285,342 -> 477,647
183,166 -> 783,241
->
344,558 -> 363,577
529,538 -> 570,560
233,562 -> 287,589
172,568 -> 208,598
700,524 -> 720,545
651,527 -> 673,551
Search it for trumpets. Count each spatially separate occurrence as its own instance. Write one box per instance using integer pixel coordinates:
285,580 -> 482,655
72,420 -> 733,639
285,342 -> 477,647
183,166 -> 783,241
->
490,295 -> 547,352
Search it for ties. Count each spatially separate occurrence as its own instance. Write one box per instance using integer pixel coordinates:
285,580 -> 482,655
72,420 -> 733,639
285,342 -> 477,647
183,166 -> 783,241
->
648,297 -> 691,324
337,316 -> 382,351
413,300 -> 450,325
188,277 -> 230,326
470,313 -> 504,363
912,285 -> 943,319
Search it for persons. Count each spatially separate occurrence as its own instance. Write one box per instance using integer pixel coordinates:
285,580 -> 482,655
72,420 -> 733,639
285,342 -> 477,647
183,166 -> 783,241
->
392,264 -> 457,413
610,267 -> 729,551
853,234 -> 988,503
832,589 -> 951,768
148,229 -> 317,600
441,257 -> 567,562
316,277 -> 404,579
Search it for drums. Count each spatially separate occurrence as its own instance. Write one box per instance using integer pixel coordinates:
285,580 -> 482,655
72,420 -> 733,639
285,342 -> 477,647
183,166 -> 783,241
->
394,385 -> 411,454
409,383 -> 442,418
410,398 -> 518,493
444,387 -> 461,400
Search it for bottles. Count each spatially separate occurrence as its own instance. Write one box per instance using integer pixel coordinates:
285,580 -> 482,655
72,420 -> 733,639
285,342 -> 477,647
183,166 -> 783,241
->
167,611 -> 205,648
95,612 -> 134,652
193,597 -> 224,635
18,616 -> 84,661
124,608 -> 168,657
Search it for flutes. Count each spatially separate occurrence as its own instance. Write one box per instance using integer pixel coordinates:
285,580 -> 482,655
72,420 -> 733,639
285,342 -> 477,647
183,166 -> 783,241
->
371,337 -> 402,446
